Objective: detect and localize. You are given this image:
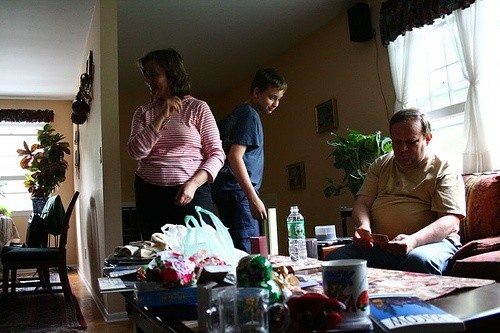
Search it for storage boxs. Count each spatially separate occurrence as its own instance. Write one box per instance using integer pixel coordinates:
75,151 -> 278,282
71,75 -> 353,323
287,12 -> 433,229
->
134,281 -> 197,308
250,236 -> 267,257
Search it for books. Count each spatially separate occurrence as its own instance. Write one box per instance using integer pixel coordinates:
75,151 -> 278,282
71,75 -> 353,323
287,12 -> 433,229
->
97,258 -> 149,293
369,293 -> 465,333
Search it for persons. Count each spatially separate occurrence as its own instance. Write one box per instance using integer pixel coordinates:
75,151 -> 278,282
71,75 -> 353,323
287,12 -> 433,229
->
326,109 -> 466,276
128,49 -> 226,241
210,68 -> 287,255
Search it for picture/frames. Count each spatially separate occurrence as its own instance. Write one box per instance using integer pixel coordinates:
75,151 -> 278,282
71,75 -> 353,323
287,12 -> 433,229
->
314,98 -> 338,134
285,161 -> 306,191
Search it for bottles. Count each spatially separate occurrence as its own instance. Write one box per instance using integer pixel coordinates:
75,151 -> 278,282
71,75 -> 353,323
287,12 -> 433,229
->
287,206 -> 307,265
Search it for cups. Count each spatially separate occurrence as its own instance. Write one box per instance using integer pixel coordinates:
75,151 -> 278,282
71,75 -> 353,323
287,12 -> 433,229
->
314,225 -> 335,241
320,259 -> 371,322
217,287 -> 291,333
294,238 -> 318,266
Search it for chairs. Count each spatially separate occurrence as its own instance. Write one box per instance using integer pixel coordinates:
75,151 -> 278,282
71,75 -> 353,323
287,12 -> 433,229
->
1,191 -> 79,303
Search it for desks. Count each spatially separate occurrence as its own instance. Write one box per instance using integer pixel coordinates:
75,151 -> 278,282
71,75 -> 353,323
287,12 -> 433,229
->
338,207 -> 353,237
0,215 -> 20,252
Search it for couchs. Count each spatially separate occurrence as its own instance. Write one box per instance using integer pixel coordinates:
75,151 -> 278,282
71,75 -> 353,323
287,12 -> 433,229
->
321,171 -> 500,283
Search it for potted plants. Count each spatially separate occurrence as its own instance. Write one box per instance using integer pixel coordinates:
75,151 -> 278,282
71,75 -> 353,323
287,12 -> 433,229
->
322,127 -> 392,200
17,124 -> 70,212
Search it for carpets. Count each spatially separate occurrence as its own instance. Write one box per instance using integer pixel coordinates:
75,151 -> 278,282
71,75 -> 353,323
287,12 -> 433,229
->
0,275 -> 87,333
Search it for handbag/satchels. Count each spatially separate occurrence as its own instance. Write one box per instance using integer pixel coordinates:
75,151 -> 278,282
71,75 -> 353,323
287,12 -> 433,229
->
151,205 -> 249,267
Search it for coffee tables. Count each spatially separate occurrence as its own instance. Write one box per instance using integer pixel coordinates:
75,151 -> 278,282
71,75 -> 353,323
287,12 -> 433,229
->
102,268 -> 500,333
